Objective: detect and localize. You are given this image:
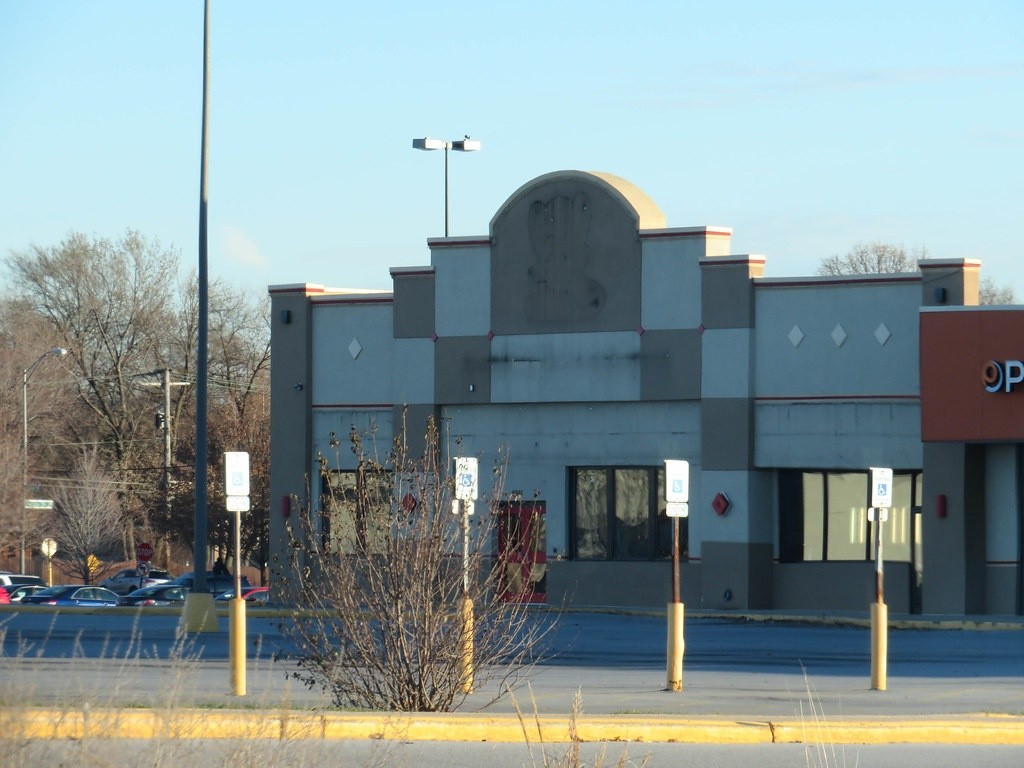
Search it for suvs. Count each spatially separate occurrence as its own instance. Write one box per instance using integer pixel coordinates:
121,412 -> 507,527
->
173,572 -> 251,595
99,567 -> 172,593
1,571 -> 47,588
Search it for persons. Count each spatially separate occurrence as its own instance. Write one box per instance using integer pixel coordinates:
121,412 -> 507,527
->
212,557 -> 231,575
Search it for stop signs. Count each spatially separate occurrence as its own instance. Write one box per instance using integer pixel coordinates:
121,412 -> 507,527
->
137,543 -> 153,561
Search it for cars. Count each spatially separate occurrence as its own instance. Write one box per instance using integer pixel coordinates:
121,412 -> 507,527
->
116,585 -> 192,607
20,585 -> 121,608
2,583 -> 49,605
215,586 -> 268,604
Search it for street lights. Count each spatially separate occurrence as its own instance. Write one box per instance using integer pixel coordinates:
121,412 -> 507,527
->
413,135 -> 482,238
20,349 -> 66,574
